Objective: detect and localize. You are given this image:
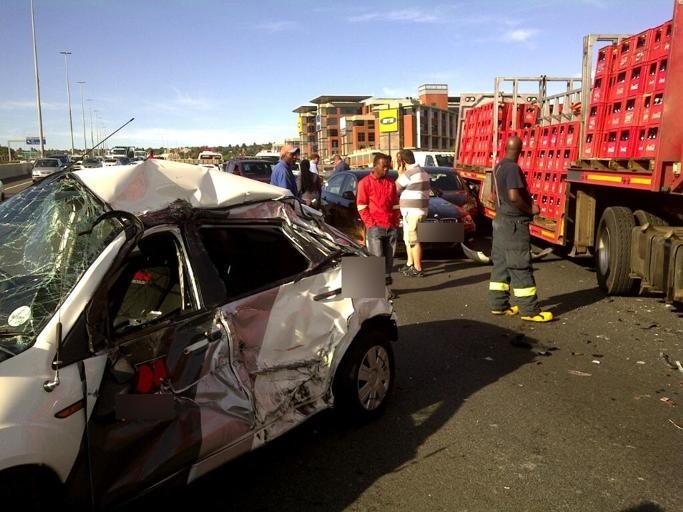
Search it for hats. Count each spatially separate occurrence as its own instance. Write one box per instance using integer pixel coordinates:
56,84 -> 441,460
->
280,145 -> 300,157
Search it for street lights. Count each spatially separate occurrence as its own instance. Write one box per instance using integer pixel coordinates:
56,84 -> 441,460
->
59,50 -> 105,157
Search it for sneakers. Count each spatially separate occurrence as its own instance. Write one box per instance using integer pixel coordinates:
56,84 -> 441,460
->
522,311 -> 554,322
399,266 -> 423,277
491,306 -> 519,316
393,264 -> 414,272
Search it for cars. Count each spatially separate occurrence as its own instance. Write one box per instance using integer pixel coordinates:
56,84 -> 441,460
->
197,148 -> 479,256
30,147 -> 147,183
0,158 -> 398,511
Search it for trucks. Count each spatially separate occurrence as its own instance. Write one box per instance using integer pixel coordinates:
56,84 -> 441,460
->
453,1 -> 683,308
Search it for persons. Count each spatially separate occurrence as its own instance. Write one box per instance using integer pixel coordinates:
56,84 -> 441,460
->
308,152 -> 319,174
332,155 -> 351,174
294,158 -> 324,212
344,153 -> 352,167
357,152 -> 399,296
143,148 -> 156,160
392,149 -> 431,278
268,144 -> 298,195
486,134 -> 555,322
